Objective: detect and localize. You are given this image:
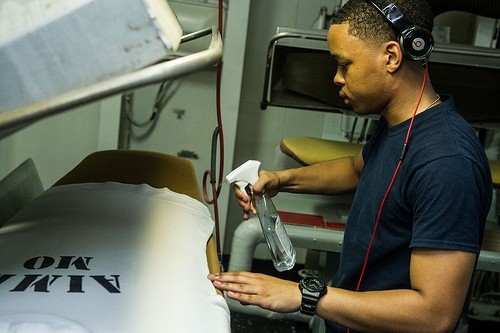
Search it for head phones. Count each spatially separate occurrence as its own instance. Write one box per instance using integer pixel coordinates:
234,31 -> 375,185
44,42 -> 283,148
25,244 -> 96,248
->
371,0 -> 435,62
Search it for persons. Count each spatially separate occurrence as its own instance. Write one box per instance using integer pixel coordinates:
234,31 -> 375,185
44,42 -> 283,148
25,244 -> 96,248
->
208,0 -> 496,332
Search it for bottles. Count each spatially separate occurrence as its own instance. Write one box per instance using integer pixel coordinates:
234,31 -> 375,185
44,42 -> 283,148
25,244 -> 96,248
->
226,159 -> 297,272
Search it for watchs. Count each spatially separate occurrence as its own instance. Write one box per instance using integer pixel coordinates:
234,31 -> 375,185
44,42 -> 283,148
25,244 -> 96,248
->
298,275 -> 328,317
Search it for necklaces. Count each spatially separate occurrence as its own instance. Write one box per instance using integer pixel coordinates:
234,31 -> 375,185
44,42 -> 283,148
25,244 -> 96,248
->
425,92 -> 440,111
